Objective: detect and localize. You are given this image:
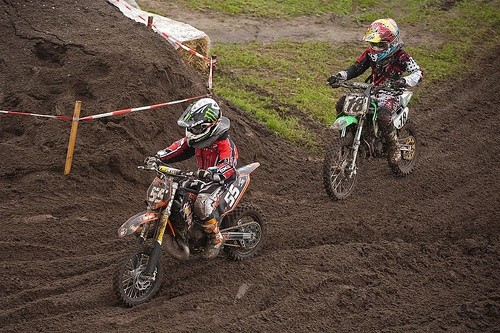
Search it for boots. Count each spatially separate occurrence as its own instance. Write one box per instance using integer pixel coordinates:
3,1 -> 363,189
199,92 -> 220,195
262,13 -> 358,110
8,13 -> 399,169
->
198,214 -> 224,260
383,126 -> 401,167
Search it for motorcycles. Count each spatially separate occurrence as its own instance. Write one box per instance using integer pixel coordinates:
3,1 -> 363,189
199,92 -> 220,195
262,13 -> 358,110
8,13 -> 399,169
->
323,75 -> 423,201
112,157 -> 269,309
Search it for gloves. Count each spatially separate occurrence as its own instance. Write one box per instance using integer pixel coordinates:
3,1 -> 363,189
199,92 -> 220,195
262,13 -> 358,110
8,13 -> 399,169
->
144,156 -> 157,165
327,74 -> 343,89
390,79 -> 405,91
195,168 -> 212,182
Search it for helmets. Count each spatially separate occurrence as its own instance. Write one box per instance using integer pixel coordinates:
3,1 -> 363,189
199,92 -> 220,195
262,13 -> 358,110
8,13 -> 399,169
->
363,17 -> 400,63
176,98 -> 223,142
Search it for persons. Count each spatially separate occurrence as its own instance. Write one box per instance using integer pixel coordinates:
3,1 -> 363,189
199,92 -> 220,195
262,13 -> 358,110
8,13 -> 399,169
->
144,97 -> 239,261
326,19 -> 421,166
